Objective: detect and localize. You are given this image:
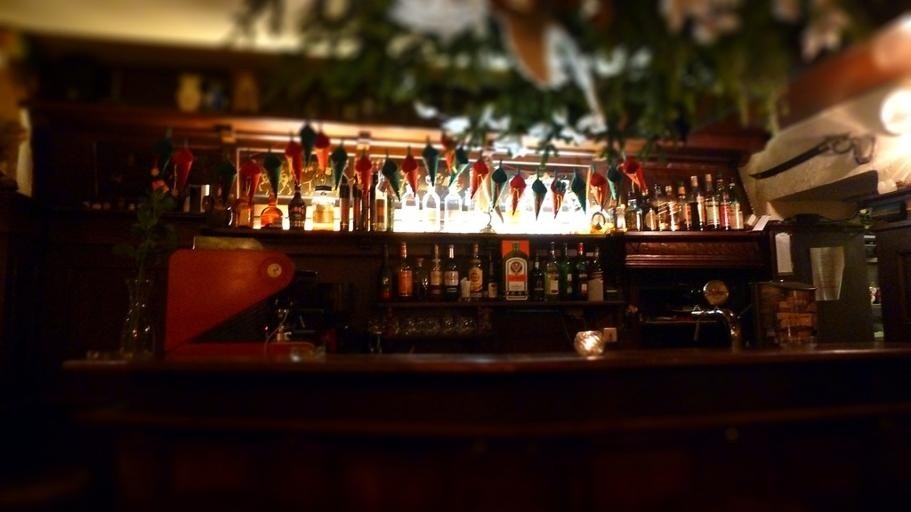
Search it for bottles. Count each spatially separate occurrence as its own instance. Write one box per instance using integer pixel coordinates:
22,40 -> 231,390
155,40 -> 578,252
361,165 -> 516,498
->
87,134 -> 747,239
370,241 -> 605,306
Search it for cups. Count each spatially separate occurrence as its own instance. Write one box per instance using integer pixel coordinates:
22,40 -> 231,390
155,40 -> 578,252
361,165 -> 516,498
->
362,313 -> 477,338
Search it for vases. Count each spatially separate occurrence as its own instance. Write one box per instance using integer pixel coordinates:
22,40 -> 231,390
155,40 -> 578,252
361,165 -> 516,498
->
120,279 -> 162,360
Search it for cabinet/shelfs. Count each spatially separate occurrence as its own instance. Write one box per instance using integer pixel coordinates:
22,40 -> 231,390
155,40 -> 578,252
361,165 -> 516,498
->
199,227 -> 772,355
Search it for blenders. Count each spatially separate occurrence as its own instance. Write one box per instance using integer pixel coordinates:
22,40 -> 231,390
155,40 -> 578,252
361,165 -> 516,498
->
318,281 -> 354,354
